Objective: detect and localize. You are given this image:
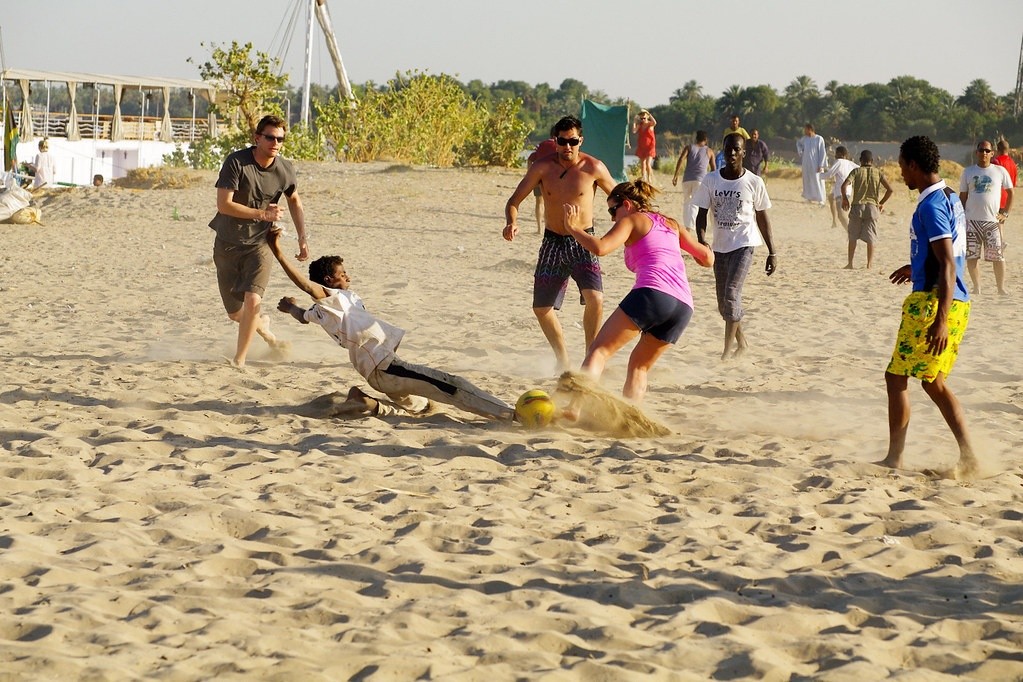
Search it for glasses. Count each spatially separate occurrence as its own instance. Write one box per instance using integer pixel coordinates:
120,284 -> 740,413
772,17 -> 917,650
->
555,135 -> 580,146
977,148 -> 991,154
639,118 -> 646,120
258,132 -> 286,143
607,202 -> 624,216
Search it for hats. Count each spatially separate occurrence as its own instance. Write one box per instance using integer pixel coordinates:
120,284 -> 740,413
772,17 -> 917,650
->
637,109 -> 648,114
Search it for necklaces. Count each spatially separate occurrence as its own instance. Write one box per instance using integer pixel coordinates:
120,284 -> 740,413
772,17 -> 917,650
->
557,160 -> 578,179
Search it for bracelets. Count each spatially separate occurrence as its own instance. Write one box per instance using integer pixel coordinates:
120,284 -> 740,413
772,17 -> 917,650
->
1001,210 -> 1008,217
769,253 -> 777,256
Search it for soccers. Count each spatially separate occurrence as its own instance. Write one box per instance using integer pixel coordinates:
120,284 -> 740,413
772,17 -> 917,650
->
515,390 -> 555,429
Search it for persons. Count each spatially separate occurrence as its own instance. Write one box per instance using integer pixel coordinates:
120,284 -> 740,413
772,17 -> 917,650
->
266,222 -> 565,424
208,116 -> 309,367
955,139 -> 1014,295
877,135 -> 984,471
527,122 -> 555,234
671,131 -> 716,237
716,149 -> 731,170
840,150 -> 894,270
723,116 -> 751,144
795,124 -> 828,206
631,109 -> 656,182
93,174 -> 105,189
502,117 -> 619,373
560,177 -> 715,407
991,141 -> 1018,252
744,128 -> 769,178
690,133 -> 778,362
820,145 -> 860,232
25,139 -> 57,189
